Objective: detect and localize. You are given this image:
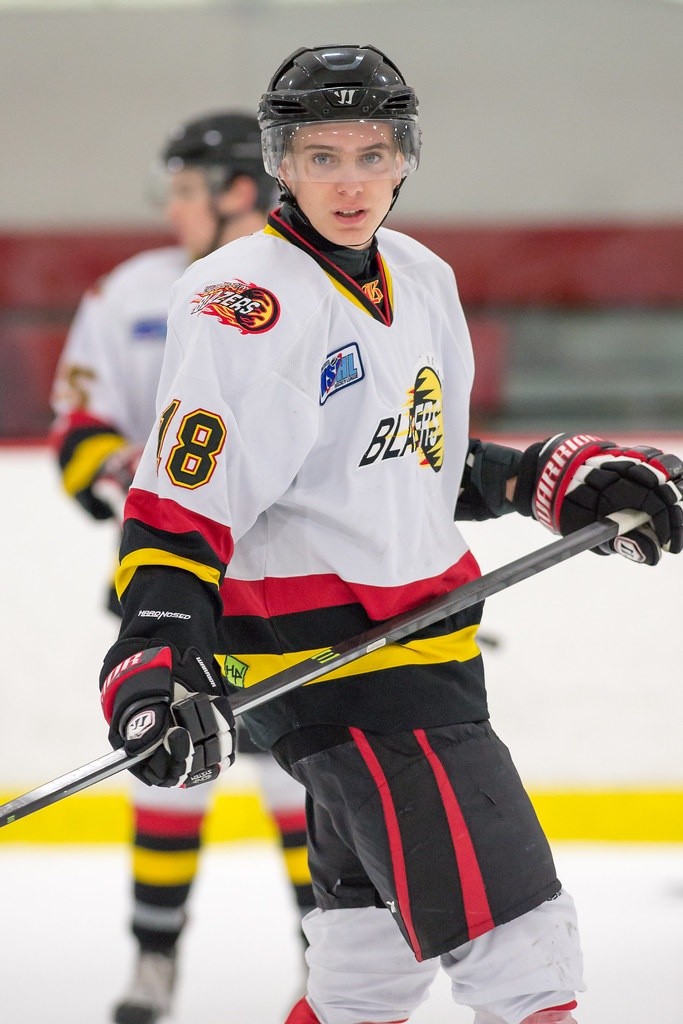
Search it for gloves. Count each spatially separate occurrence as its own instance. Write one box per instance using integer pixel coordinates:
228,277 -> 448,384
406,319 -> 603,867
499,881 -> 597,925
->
513,431 -> 683,567
92,447 -> 143,530
98,572 -> 239,791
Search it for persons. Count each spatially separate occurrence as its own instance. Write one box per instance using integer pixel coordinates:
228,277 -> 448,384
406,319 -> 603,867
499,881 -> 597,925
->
99,45 -> 683,1024
47,110 -> 321,1024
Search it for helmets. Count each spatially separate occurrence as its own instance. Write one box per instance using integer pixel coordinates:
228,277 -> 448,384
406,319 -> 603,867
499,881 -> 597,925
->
257,42 -> 423,205
163,112 -> 278,192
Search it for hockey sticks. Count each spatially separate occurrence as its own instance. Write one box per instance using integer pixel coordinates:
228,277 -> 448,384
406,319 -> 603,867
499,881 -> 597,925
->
0,506 -> 651,826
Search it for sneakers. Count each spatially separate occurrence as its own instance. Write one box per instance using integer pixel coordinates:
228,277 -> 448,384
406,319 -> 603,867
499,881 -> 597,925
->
113,923 -> 183,1024
